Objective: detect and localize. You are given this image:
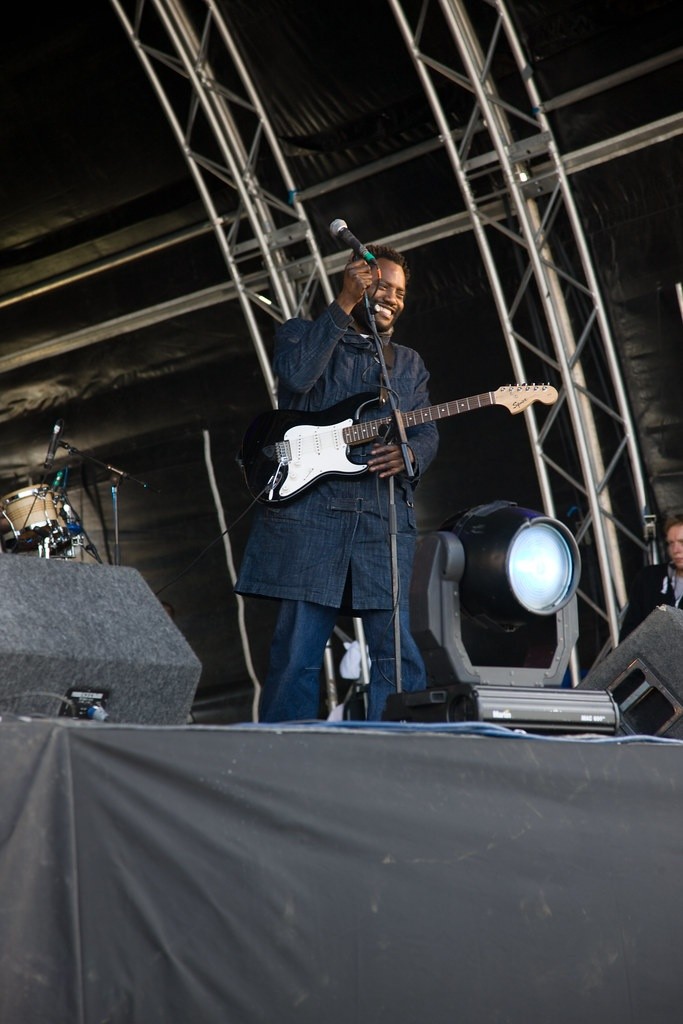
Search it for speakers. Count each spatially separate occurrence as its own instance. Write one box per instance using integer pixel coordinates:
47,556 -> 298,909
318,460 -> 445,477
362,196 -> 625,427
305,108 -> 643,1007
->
0,552 -> 202,728
576,604 -> 683,744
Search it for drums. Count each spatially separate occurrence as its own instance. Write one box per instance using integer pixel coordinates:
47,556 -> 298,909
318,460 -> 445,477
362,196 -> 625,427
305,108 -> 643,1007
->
1,481 -> 73,555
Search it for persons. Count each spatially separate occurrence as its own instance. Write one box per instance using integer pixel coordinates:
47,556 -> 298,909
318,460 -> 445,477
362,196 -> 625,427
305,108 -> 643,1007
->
234,245 -> 439,723
610,510 -> 683,738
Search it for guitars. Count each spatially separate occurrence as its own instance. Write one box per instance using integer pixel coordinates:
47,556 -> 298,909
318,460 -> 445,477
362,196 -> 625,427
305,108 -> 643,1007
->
238,381 -> 564,503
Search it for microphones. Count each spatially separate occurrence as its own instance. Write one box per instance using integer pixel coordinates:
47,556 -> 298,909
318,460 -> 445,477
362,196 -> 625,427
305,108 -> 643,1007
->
43,419 -> 62,469
330,218 -> 378,266
51,468 -> 65,492
